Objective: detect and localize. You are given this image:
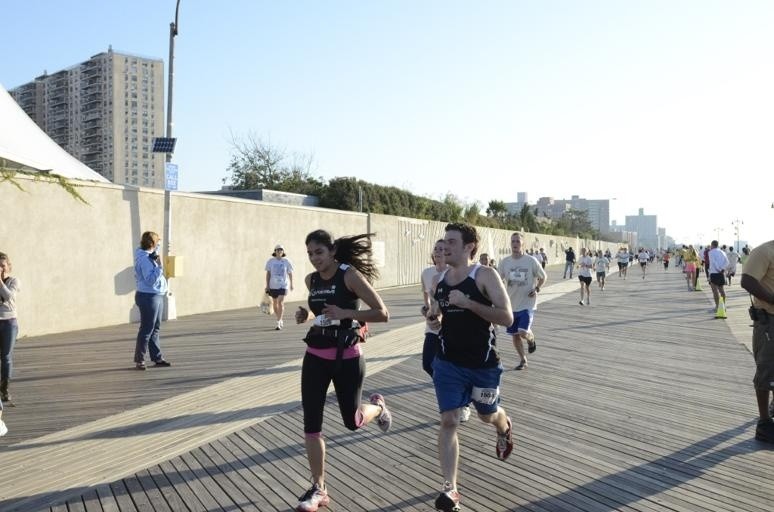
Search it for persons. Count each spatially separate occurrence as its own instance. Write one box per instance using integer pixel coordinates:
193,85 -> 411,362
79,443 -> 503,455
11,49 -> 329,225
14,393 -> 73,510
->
425,221 -> 513,512
526,240 -> 737,313
479,253 -> 491,265
736,244 -> 753,264
265,245 -> 295,329
134,231 -> 172,370
739,239 -> 774,443
0,252 -> 20,401
498,231 -> 547,370
294,228 -> 393,512
489,260 -> 497,270
420,238 -> 472,422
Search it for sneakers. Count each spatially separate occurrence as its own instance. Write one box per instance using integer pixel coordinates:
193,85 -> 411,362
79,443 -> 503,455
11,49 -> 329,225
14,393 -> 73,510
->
496,416 -> 513,460
529,342 -> 536,352
434,481 -> 460,512
276,325 -> 280,330
371,393 -> 392,431
155,359 -> 171,366
136,361 -> 146,370
516,362 -> 528,369
1,388 -> 10,401
460,406 -> 470,421
580,301 -> 584,305
755,418 -> 774,442
296,482 -> 329,512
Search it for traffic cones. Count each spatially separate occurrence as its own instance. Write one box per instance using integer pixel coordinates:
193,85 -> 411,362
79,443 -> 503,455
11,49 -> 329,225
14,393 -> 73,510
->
715,296 -> 727,319
695,279 -> 704,291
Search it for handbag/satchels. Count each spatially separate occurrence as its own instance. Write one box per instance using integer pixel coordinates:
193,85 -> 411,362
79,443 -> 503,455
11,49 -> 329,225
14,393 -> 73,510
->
306,322 -> 368,348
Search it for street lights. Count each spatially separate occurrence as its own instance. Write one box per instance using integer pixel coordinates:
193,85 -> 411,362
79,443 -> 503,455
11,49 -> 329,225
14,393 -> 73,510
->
598,198 -> 616,249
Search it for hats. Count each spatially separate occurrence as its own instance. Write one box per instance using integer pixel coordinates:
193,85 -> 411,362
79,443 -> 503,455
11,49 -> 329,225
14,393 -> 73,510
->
275,244 -> 283,249
